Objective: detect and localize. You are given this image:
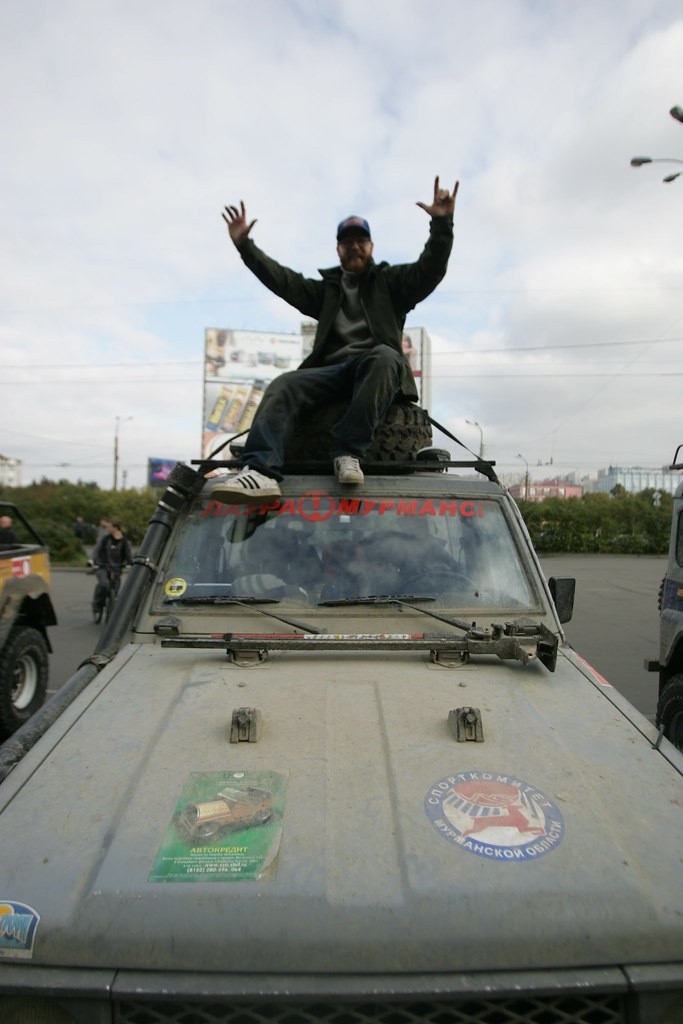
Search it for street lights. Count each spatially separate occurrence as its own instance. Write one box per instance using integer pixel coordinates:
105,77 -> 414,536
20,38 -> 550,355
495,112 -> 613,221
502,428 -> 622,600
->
112,417 -> 135,495
517,455 -> 529,507
464,419 -> 484,483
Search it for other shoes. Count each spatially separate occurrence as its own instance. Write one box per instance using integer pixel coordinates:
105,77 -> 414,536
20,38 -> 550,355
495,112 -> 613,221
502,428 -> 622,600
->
92,603 -> 101,613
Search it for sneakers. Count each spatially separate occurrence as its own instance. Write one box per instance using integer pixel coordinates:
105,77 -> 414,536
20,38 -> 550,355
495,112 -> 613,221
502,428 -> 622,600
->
334,455 -> 364,483
211,465 -> 281,504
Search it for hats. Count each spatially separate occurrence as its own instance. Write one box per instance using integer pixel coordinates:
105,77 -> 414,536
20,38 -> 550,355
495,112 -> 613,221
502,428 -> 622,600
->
337,217 -> 370,237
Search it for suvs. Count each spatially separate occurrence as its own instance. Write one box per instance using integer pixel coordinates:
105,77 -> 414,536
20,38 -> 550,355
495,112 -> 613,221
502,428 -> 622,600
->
0,502 -> 63,742
642,442 -> 683,756
0,423 -> 683,1024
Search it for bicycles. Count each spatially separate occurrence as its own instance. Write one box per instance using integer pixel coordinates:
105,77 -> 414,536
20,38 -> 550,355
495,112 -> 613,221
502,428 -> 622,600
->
83,560 -> 133,625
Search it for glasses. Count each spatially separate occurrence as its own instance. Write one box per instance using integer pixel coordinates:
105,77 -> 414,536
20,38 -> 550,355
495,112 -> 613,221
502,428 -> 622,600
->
338,237 -> 370,247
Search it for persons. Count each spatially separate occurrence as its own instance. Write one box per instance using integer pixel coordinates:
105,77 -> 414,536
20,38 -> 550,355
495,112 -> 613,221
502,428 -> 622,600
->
212,176 -> 460,503
313,539 -> 375,596
86,516 -> 134,614
0,516 -> 19,544
